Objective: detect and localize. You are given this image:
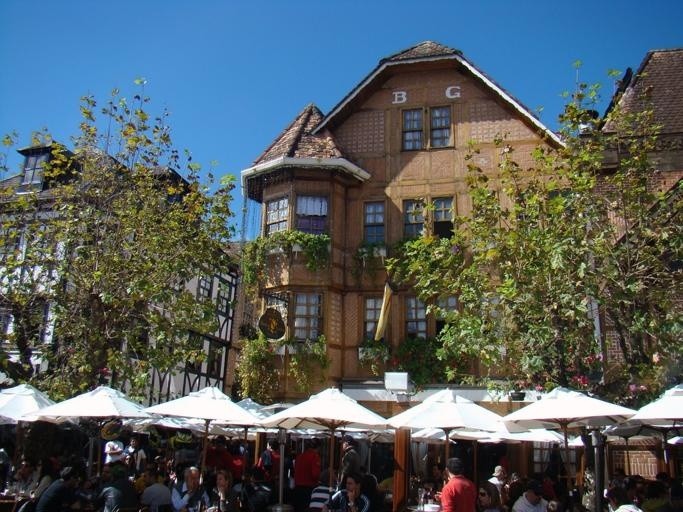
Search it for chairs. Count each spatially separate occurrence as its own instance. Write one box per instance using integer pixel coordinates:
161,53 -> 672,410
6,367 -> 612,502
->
0,462 -> 682,512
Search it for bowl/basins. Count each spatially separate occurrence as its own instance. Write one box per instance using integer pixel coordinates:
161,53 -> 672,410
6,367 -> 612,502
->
423,503 -> 441,512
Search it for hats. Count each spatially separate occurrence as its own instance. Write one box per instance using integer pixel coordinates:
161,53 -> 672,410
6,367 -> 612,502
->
528,481 -> 543,494
493,466 -> 502,476
101,422 -> 123,441
339,436 -> 353,442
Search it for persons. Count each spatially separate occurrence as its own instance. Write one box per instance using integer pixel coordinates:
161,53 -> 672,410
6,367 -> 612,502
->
0,435 -> 683,512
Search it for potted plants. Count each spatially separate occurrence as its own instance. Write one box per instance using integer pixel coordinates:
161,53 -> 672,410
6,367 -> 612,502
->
509,382 -> 525,400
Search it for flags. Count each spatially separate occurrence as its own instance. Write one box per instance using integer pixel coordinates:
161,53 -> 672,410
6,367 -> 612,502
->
374,284 -> 393,341
